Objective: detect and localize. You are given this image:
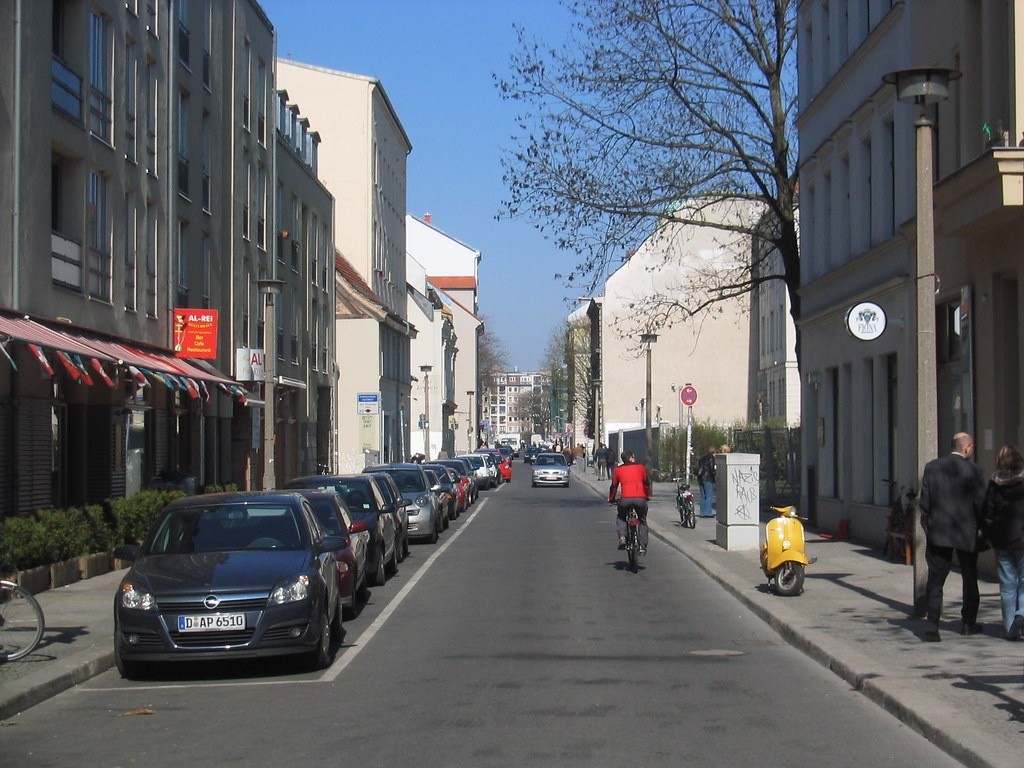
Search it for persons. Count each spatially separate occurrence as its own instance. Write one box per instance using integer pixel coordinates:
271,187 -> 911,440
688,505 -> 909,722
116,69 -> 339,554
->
412,453 -> 421,465
608,451 -> 650,553
719,444 -> 730,453
480,443 -> 487,449
982,441 -> 1024,640
593,444 -> 616,481
696,447 -> 716,518
918,432 -> 990,642
564,443 -> 585,465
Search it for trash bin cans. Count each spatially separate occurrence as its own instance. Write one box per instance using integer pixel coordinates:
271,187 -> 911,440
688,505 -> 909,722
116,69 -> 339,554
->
577,456 -> 585,472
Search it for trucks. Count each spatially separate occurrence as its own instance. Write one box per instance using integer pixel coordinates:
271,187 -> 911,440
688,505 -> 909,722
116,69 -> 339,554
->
498,433 -> 520,458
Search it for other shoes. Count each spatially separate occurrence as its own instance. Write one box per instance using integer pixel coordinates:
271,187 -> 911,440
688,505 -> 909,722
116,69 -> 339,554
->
1008,616 -> 1024,640
617,538 -> 626,551
962,624 -> 983,635
926,631 -> 941,642
638,546 -> 646,554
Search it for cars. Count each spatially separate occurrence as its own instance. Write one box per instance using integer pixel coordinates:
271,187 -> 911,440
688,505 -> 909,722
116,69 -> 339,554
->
531,453 -> 571,488
286,474 -> 398,587
361,463 -> 441,544
524,448 -> 548,465
420,446 -> 514,533
364,473 -> 413,563
113,489 -> 348,680
260,489 -> 370,622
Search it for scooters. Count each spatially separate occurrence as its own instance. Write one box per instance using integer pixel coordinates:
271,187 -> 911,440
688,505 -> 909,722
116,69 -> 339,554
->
759,504 -> 810,597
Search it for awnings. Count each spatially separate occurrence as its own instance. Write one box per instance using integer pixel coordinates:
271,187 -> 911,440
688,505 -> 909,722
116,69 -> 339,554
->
0,311 -> 244,386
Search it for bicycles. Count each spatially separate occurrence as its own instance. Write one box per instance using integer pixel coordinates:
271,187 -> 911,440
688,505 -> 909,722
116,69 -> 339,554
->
672,476 -> 696,529
0,579 -> 46,663
608,497 -> 650,574
882,477 -> 917,559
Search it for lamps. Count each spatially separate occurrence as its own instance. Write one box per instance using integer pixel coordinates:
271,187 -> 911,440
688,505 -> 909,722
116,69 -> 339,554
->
276,415 -> 297,425
117,409 -> 132,417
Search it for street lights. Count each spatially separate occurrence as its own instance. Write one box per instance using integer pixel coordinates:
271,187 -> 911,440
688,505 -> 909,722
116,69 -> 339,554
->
589,379 -> 603,476
466,390 -> 476,454
884,66 -> 963,620
252,278 -> 288,492
638,333 -> 661,479
570,399 -> 577,448
416,364 -> 435,463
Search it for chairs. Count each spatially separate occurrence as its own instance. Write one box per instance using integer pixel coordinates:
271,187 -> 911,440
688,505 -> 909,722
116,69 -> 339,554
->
885,515 -> 913,565
314,504 -> 341,534
539,459 -> 547,464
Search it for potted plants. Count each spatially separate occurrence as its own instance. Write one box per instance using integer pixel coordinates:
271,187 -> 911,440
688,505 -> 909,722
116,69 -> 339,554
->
0,490 -> 188,602
